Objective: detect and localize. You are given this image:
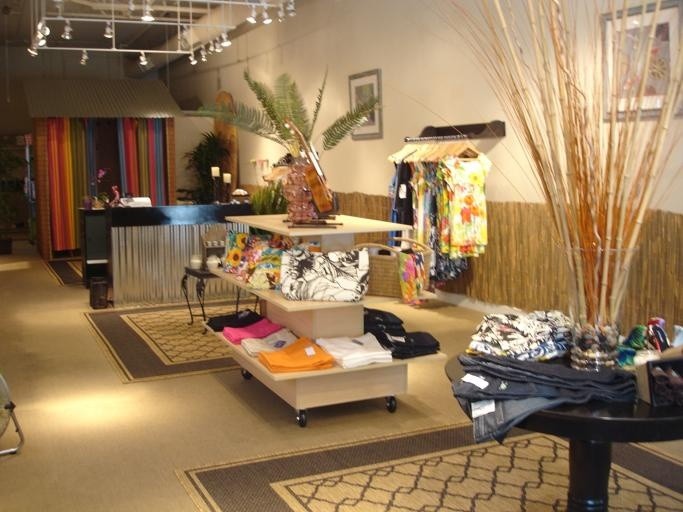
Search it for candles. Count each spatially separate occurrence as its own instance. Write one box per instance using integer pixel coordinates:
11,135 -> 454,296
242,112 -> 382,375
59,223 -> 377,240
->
223,173 -> 231,183
211,166 -> 220,177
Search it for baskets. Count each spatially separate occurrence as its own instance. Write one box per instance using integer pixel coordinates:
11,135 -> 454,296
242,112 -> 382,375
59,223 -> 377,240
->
353,237 -> 433,298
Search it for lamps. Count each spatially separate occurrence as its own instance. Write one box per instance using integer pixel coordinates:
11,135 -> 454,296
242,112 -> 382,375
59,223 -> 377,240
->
22,0 -> 299,67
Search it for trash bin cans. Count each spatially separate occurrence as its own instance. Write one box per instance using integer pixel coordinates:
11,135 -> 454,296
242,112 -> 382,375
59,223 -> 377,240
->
89,275 -> 109,310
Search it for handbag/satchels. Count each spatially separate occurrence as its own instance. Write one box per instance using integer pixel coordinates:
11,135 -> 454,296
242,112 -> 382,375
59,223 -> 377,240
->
279,247 -> 370,301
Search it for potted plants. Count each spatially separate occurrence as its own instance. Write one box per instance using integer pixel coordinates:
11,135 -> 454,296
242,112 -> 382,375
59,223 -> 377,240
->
179,68 -> 382,224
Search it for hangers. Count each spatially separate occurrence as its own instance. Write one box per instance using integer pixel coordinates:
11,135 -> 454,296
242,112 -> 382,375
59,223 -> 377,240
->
386,133 -> 485,164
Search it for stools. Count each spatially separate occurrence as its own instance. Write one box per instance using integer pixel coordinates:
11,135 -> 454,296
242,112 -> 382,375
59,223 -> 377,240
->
181,265 -> 260,334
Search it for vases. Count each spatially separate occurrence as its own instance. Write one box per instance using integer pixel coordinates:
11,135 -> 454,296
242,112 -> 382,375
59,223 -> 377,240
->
556,245 -> 640,373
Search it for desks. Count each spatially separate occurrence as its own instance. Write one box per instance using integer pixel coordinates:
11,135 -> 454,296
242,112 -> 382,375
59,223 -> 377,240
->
77,202 -> 254,305
443,351 -> 683,511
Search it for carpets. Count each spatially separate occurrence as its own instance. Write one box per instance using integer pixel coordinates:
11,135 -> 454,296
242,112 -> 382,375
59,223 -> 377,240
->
80,297 -> 262,384
174,418 -> 683,512
40,258 -> 82,287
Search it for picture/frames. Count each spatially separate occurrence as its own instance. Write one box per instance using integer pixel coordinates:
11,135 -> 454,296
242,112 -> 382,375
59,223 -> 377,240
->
348,68 -> 384,141
599,0 -> 683,123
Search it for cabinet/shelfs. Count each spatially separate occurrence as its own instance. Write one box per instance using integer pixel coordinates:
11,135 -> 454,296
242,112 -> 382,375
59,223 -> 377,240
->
200,214 -> 414,429
